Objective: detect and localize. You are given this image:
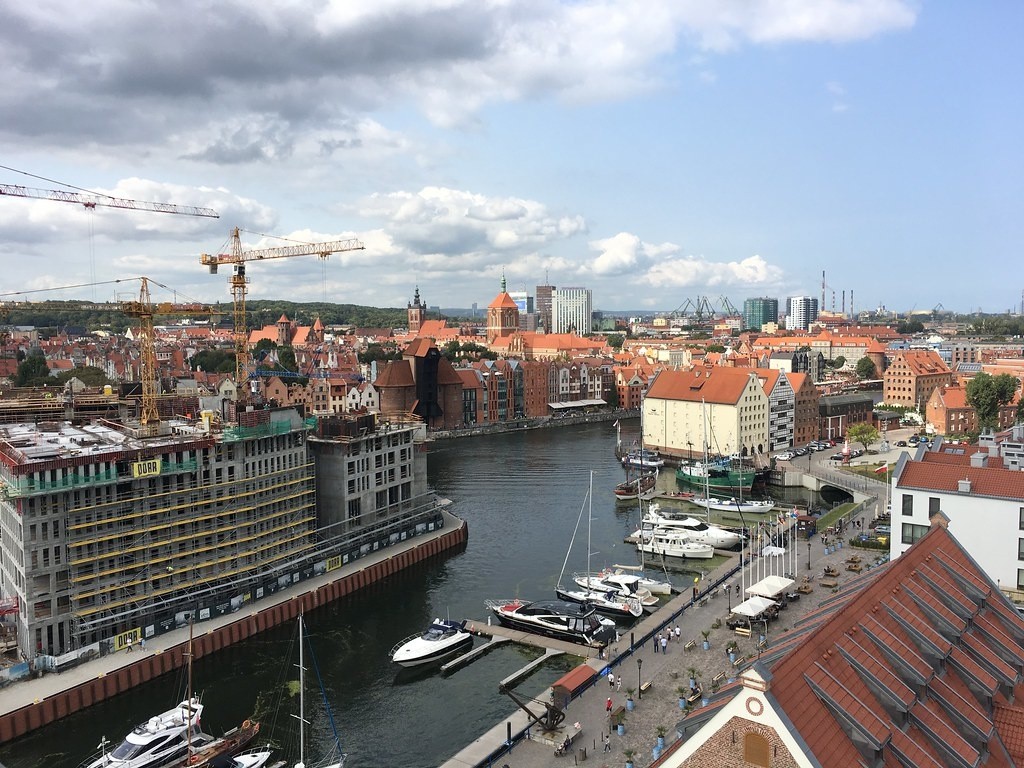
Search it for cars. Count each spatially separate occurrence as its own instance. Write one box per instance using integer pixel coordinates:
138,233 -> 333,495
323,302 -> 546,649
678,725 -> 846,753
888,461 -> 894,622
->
773,435 -> 864,462
892,433 -> 972,448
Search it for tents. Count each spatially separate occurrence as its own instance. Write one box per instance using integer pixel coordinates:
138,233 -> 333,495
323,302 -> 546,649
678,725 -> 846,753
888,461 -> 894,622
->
745,575 -> 796,599
732,596 -> 776,617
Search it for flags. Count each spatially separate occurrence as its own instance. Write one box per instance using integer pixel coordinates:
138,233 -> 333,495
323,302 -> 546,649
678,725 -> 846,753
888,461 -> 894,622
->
741,507 -> 801,542
875,463 -> 888,474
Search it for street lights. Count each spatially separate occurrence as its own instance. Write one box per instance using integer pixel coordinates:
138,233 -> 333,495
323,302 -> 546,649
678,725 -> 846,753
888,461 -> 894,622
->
725,584 -> 732,615
637,658 -> 642,700
807,543 -> 812,571
860,516 -> 866,536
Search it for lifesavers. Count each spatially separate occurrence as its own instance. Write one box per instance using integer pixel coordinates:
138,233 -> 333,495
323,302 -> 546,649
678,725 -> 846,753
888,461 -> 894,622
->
242,720 -> 250,729
255,723 -> 259,730
190,756 -> 198,762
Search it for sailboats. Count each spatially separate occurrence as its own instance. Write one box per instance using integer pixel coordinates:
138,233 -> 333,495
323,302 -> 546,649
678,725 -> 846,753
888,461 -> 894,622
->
75,610 -> 348,768
553,395 -> 775,618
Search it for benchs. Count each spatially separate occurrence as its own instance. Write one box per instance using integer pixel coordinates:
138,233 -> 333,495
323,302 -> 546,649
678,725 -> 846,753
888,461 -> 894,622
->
708,586 -> 719,599
733,626 -> 752,640
684,639 -> 697,652
756,638 -> 767,650
698,597 -> 708,607
712,670 -> 727,684
847,557 -> 861,563
845,566 -> 862,573
794,587 -> 813,595
640,680 -> 654,694
824,571 -> 840,577
819,580 -> 837,588
732,656 -> 745,671
687,683 -> 703,706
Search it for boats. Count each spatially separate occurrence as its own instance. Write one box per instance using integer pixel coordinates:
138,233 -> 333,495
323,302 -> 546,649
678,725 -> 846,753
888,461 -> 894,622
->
484,597 -> 617,649
388,605 -> 475,670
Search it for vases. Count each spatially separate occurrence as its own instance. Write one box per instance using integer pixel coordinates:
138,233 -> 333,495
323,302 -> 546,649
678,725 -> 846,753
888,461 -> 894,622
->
702,699 -> 709,707
652,746 -> 661,761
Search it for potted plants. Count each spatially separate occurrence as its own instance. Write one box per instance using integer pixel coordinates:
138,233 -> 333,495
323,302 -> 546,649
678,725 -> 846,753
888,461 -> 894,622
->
623,687 -> 637,711
760,625 -> 766,643
686,665 -> 704,688
622,748 -> 637,768
824,538 -> 835,555
865,551 -> 890,571
724,640 -> 739,663
674,685 -> 690,710
701,630 -> 711,651
836,536 -> 845,549
614,711 -> 626,736
654,724 -> 669,749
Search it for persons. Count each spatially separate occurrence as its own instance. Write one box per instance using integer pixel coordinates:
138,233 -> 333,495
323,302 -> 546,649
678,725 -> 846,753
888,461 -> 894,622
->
786,517 -> 861,543
606,698 -> 613,717
608,672 -> 614,691
126,636 -> 148,653
653,625 -> 681,655
559,719 -> 582,756
597,644 -> 609,660
771,573 -> 807,611
733,605 -> 780,640
722,582 -> 741,597
616,675 -> 621,693
689,684 -> 701,702
602,735 -> 611,754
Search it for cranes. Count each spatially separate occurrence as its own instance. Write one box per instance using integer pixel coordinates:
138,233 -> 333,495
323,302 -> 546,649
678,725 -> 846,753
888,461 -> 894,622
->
0,276 -> 230,426
198,225 -> 367,394
0,166 -> 221,220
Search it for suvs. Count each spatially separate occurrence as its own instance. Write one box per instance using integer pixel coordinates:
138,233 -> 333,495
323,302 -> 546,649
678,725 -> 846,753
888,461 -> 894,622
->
874,524 -> 892,535
